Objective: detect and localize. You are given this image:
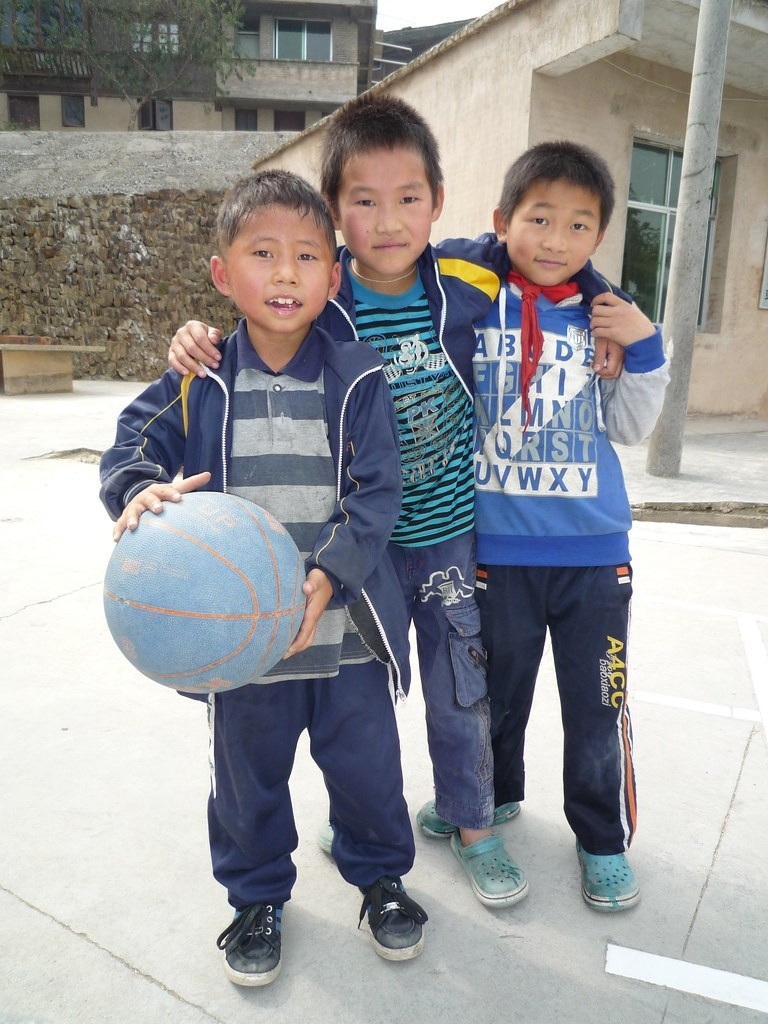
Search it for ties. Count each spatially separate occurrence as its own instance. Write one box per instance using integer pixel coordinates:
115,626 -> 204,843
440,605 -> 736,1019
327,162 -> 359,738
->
506,271 -> 577,434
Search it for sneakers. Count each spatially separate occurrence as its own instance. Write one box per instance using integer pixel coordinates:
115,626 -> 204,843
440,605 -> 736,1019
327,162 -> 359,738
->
358,876 -> 428,961
215,903 -> 284,987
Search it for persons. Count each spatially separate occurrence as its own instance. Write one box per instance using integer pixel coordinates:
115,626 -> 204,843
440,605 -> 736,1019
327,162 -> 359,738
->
417,142 -> 668,910
100,170 -> 428,988
165,90 -> 623,907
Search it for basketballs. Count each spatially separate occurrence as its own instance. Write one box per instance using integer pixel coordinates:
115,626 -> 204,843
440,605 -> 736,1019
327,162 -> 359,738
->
104,489 -> 309,697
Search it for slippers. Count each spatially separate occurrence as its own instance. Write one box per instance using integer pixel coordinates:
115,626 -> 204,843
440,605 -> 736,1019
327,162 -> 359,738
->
318,819 -> 334,853
450,827 -> 530,907
575,836 -> 640,911
415,799 -> 521,838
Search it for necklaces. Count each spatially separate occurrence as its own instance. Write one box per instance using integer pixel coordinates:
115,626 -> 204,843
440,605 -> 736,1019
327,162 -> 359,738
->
351,258 -> 417,283
